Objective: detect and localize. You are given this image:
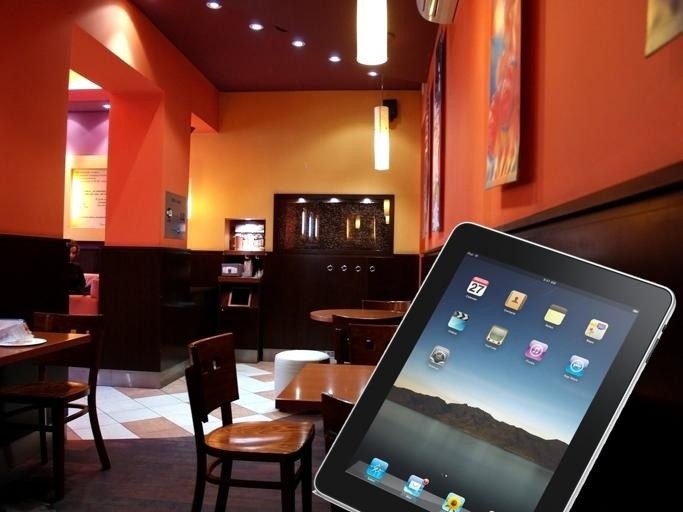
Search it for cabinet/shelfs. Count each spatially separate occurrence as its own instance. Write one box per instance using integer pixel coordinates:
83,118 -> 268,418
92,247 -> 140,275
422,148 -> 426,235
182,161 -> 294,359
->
219,299 -> 270,363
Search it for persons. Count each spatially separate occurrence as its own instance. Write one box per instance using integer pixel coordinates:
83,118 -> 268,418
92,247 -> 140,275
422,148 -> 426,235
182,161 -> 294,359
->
70,241 -> 87,294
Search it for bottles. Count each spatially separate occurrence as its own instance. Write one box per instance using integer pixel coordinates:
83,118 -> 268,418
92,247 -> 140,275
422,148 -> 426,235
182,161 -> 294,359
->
243,259 -> 253,276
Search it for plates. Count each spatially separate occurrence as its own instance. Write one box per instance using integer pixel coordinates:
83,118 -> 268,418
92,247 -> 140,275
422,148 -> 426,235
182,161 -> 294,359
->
0,338 -> 47,347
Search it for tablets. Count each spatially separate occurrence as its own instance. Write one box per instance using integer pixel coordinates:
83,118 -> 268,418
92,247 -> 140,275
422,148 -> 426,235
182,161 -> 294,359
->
313,221 -> 677,511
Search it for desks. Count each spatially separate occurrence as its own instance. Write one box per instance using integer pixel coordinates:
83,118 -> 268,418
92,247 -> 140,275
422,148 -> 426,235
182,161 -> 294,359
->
276,361 -> 376,409
0,333 -> 90,366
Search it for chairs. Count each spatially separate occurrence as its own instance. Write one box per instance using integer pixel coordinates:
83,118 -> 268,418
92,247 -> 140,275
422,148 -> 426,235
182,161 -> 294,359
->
320,390 -> 353,456
188,331 -> 315,510
307,300 -> 409,366
0,313 -> 110,501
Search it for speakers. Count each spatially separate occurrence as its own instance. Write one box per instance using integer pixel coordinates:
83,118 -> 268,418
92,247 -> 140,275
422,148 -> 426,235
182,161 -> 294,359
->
383,99 -> 398,122
